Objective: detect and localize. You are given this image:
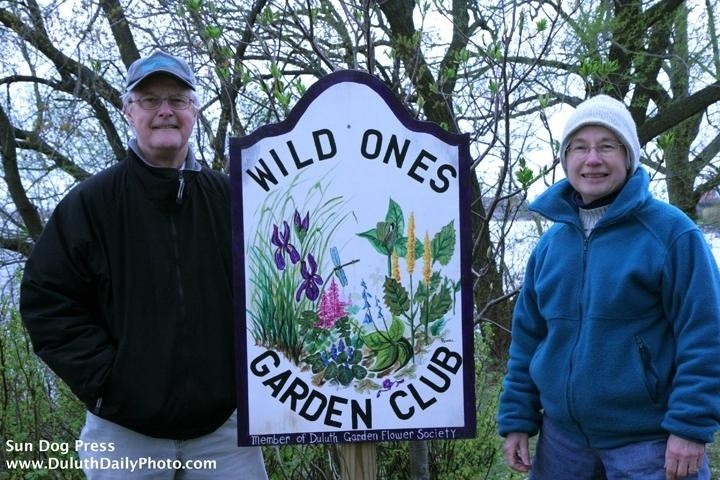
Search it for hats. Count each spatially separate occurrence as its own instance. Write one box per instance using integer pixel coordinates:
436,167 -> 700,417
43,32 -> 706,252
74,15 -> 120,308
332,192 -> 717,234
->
559,92 -> 641,183
124,49 -> 197,92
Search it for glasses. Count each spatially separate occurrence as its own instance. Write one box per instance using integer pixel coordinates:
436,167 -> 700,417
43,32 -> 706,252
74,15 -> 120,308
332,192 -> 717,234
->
126,94 -> 194,110
564,141 -> 624,159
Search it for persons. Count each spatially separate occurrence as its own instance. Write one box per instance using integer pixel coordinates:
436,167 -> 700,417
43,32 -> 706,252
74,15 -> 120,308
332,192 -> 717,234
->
19,43 -> 276,480
496,90 -> 720,480
